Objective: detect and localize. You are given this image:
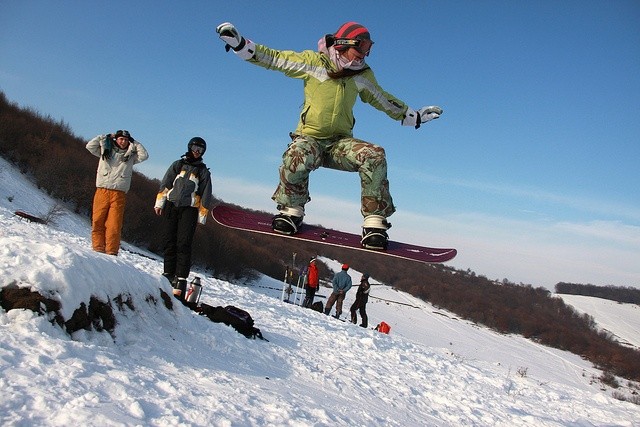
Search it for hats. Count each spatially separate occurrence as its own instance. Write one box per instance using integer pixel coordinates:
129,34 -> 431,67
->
342,264 -> 349,270
309,256 -> 316,263
115,130 -> 130,138
335,21 -> 370,52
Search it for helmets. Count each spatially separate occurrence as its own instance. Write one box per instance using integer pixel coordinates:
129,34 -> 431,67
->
188,137 -> 206,151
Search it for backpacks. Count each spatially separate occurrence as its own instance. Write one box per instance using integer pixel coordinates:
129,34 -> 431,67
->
201,303 -> 263,341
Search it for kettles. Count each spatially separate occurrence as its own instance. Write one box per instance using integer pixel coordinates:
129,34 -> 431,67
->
186,277 -> 203,306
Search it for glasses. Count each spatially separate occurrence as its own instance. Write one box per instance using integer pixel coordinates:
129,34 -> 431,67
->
189,143 -> 204,154
333,36 -> 374,56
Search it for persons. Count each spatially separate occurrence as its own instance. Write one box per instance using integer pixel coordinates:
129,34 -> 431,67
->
302,258 -> 319,307
86,131 -> 149,255
215,22 -> 443,251
153,138 -> 212,289
324,264 -> 352,319
350,273 -> 370,328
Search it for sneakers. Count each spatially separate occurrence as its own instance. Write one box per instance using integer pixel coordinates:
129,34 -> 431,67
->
271,214 -> 302,234
362,228 -> 389,250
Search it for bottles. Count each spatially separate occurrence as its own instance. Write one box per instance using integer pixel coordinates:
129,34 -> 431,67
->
175,277 -> 187,300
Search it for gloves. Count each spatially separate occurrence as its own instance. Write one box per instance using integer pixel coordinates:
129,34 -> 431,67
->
216,21 -> 256,61
401,106 -> 443,127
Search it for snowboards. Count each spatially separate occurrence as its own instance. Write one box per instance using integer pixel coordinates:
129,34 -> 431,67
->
212,202 -> 457,264
